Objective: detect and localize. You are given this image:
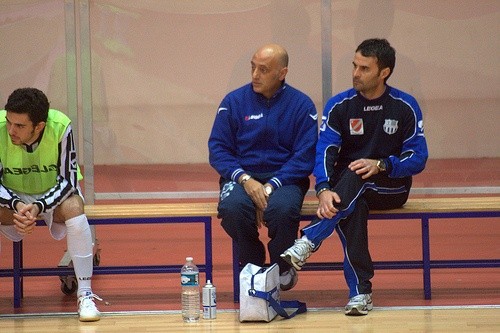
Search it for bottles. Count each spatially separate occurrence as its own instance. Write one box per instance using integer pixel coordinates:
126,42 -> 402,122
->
202,280 -> 217,319
180,256 -> 201,323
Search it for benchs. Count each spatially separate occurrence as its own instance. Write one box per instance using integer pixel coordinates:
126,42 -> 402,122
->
233,197 -> 500,300
0,202 -> 219,308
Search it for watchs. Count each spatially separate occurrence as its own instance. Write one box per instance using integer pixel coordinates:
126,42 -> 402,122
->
264,185 -> 273,196
377,160 -> 386,171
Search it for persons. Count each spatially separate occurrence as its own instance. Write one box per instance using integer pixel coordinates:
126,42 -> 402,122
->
0,87 -> 100,322
279,39 -> 429,316
207,41 -> 318,292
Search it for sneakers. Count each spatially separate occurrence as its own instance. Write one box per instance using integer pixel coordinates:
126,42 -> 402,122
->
77,291 -> 103,322
280,239 -> 312,270
344,293 -> 372,316
278,266 -> 299,291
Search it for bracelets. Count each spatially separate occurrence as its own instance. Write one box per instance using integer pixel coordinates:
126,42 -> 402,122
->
316,188 -> 326,197
241,175 -> 251,185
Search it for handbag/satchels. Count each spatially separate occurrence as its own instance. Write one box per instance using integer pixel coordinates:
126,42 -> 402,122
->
239,262 -> 306,322
